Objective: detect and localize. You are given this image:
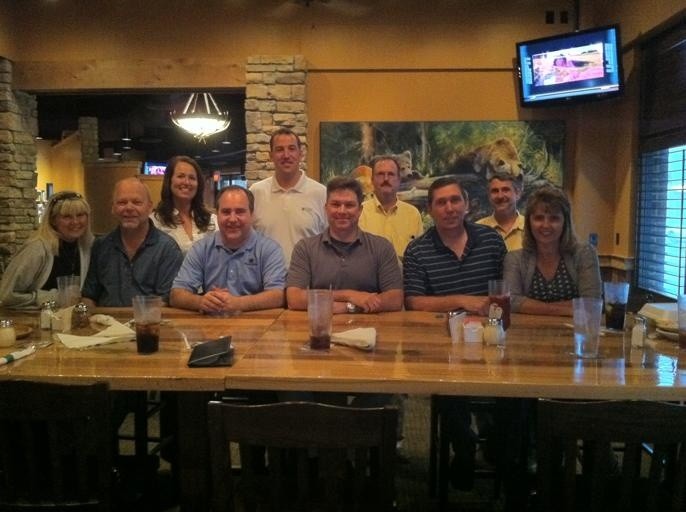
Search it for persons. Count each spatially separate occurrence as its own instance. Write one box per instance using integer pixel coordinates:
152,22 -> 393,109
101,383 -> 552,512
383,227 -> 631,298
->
169,185 -> 288,314
474,175 -> 525,251
402,178 -> 507,316
0,190 -> 95,311
502,186 -> 603,316
80,177 -> 184,307
149,156 -> 220,296
248,128 -> 329,273
358,157 -> 424,278
285,176 -> 405,314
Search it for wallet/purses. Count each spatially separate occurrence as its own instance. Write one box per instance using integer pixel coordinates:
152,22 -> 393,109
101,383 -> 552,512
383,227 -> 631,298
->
188,335 -> 233,366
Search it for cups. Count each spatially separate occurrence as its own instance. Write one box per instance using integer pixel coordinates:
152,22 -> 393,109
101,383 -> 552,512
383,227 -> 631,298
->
132,295 -> 162,355
488,280 -> 511,335
572,297 -> 603,358
308,289 -> 331,350
678,294 -> 686,349
603,281 -> 630,331
65,276 -> 81,309
57,277 -> 74,309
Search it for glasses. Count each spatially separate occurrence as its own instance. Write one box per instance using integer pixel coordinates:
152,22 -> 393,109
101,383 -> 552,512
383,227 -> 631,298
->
49,192 -> 81,209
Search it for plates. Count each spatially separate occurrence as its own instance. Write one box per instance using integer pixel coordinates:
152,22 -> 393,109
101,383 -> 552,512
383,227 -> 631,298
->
13,325 -> 33,339
655,327 -> 678,340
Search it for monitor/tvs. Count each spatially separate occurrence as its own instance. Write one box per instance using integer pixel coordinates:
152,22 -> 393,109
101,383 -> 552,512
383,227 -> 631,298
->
141,161 -> 168,176
515,23 -> 625,108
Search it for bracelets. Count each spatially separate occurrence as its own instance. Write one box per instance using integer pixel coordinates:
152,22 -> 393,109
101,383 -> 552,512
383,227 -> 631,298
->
347,302 -> 356,313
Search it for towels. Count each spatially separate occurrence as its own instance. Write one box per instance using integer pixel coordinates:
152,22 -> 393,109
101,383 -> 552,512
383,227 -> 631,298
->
56,321 -> 136,351
331,327 -> 377,350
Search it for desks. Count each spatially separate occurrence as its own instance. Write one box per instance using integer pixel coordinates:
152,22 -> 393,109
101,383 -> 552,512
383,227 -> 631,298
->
0,305 -> 285,511
226,310 -> 685,512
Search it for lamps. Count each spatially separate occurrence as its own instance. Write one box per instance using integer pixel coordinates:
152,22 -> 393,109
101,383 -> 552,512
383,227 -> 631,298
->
169,92 -> 232,144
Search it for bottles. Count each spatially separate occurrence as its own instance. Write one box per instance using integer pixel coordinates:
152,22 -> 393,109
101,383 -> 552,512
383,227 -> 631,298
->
640,317 -> 647,345
631,317 -> 644,348
40,300 -> 89,330
0,319 -> 16,347
448,311 -> 506,345
